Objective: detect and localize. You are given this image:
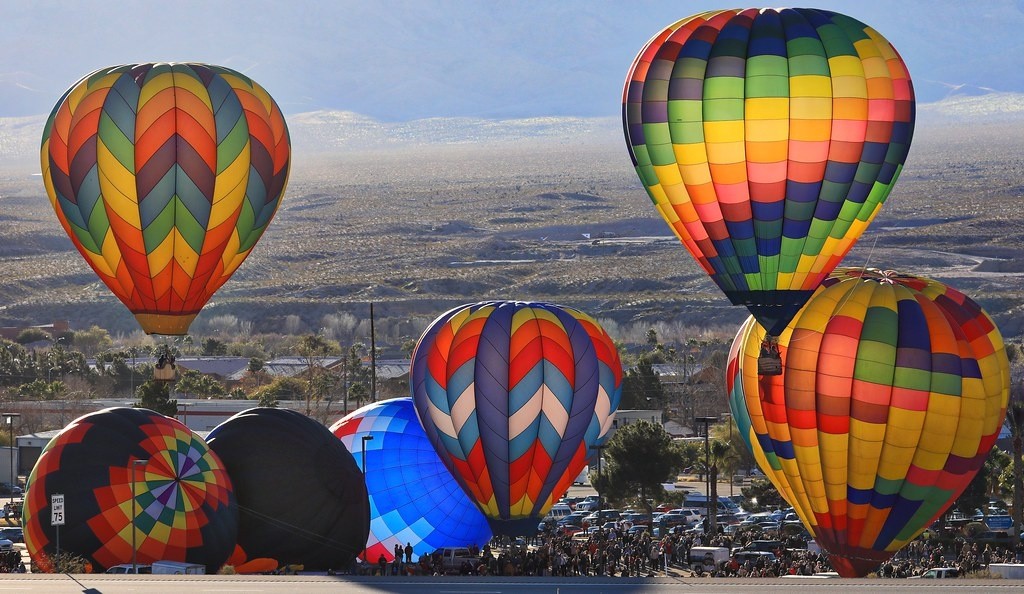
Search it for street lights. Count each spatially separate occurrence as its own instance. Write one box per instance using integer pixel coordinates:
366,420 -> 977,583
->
176,402 -> 194,428
360,434 -> 372,566
130,459 -> 148,572
588,442 -> 608,576
1,412 -> 21,502
693,414 -> 720,533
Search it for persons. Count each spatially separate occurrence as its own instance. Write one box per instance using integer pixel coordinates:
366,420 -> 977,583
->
770,345 -> 780,359
0,477 -> 1021,583
759,343 -> 768,358
157,353 -> 175,371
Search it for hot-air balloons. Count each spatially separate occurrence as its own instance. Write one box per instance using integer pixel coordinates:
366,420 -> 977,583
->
410,300 -> 623,556
20,407 -> 234,576
326,397 -> 495,567
39,61 -> 295,381
620,7 -> 917,375
725,263 -> 1013,582
201,406 -> 371,576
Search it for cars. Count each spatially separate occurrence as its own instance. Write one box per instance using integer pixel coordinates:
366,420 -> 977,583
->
0,478 -> 1022,577
